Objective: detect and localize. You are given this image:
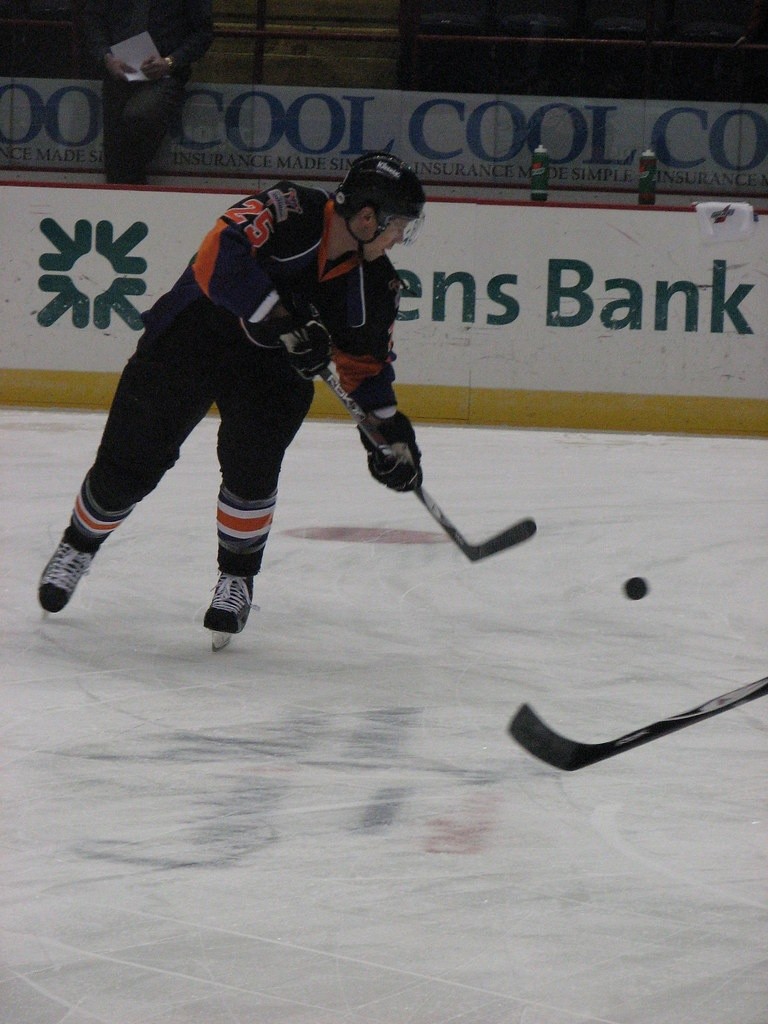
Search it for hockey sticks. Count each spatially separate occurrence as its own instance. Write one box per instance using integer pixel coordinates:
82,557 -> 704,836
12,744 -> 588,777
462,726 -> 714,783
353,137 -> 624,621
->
296,331 -> 541,566
506,676 -> 768,774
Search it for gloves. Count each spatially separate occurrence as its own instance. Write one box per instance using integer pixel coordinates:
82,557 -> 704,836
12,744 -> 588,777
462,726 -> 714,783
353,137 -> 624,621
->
356,409 -> 423,493
264,298 -> 332,379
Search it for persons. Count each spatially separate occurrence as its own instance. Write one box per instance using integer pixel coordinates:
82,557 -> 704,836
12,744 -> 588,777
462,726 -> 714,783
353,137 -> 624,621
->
80,0 -> 215,185
40,152 -> 425,633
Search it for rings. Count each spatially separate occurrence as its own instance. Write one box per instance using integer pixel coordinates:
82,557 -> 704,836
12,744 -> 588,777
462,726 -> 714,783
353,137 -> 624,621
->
155,69 -> 158,72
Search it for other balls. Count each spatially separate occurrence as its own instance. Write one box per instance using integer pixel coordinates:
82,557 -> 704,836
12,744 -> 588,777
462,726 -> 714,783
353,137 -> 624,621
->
625,577 -> 648,601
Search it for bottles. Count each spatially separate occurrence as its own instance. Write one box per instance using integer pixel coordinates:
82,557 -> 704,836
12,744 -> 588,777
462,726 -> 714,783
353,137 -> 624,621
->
530,144 -> 549,201
639,149 -> 657,205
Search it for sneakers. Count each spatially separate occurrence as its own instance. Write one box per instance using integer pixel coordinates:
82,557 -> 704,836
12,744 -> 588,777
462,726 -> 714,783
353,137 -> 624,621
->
203,571 -> 260,652
38,515 -> 100,621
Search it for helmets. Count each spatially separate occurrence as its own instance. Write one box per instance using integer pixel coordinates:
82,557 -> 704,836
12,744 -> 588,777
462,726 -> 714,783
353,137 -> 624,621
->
334,151 -> 426,237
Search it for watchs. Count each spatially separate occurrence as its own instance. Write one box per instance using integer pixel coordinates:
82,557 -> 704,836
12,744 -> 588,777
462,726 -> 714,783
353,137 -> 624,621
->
165,57 -> 175,71
